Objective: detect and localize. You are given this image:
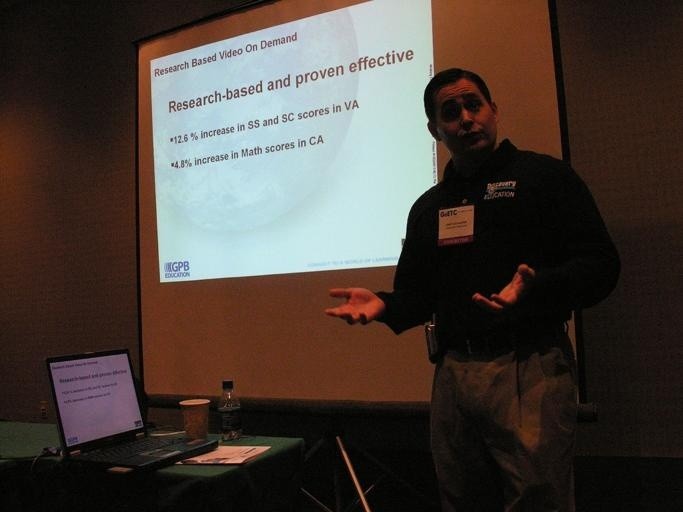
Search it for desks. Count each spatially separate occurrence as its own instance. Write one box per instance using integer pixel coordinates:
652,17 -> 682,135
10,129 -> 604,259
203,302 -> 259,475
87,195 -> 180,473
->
0,418 -> 307,511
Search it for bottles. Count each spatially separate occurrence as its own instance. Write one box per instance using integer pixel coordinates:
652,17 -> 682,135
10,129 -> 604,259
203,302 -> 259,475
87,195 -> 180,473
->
218,380 -> 240,441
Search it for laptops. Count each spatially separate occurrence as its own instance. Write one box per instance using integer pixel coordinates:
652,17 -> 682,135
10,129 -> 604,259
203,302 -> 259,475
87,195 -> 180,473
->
46,348 -> 218,473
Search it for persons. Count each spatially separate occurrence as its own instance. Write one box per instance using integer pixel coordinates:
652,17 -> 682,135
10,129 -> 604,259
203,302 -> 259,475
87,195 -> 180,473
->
325,68 -> 621,512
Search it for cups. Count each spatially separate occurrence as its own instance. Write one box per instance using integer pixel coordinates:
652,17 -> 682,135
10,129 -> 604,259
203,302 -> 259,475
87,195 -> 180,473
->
179,399 -> 210,440
